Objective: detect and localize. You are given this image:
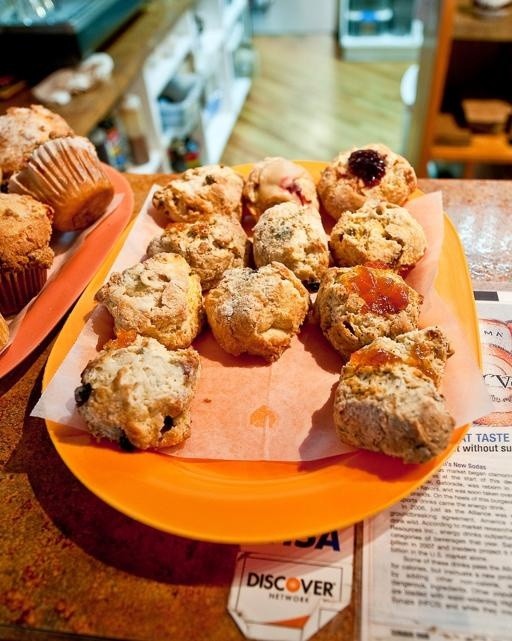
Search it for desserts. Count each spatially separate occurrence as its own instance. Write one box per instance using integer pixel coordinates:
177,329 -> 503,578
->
0,105 -> 453,465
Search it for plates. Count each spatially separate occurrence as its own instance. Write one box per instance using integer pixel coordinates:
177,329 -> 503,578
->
1,163 -> 136,382
40,157 -> 491,548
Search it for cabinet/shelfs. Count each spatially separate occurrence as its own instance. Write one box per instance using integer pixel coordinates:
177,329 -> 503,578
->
0,1 -> 257,174
405,0 -> 512,183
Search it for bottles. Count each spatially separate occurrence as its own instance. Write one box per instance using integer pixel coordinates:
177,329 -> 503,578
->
97,95 -> 150,170
